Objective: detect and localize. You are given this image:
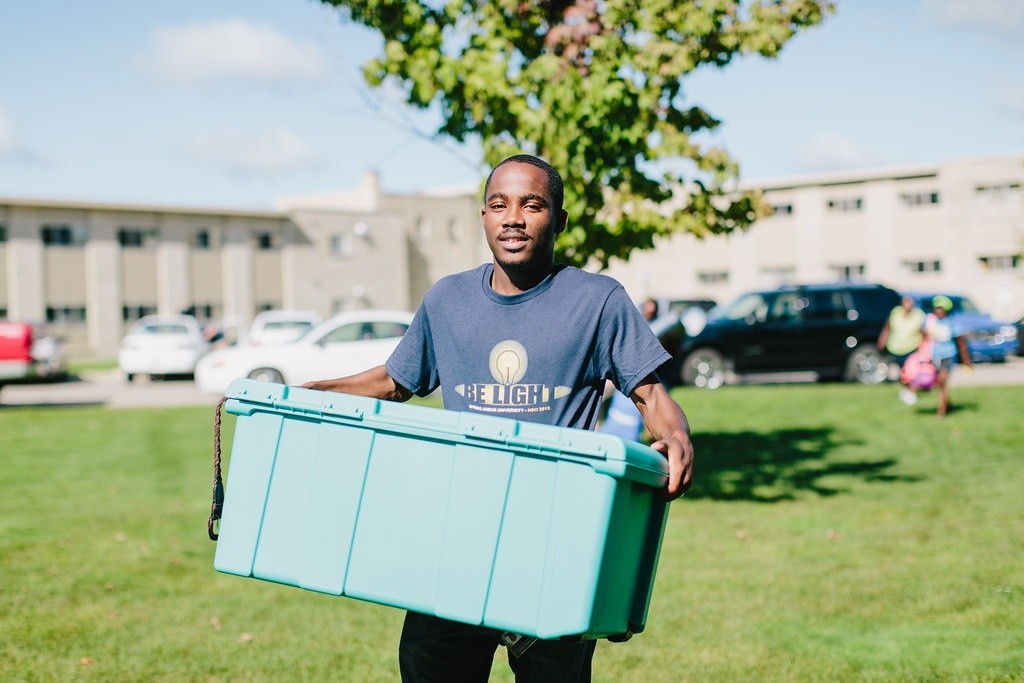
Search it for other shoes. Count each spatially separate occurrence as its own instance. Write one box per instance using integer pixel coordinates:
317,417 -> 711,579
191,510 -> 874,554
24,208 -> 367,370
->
899,386 -> 917,407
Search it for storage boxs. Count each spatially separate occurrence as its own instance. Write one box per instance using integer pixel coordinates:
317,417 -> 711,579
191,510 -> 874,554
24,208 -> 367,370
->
207,379 -> 672,644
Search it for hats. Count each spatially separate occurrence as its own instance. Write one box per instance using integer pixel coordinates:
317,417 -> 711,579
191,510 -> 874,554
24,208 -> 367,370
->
933,296 -> 952,311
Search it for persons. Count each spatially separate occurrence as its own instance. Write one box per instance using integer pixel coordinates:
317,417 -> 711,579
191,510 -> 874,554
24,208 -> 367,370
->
878,294 -> 972,415
640,298 -> 658,321
294,155 -> 694,683
594,378 -> 642,442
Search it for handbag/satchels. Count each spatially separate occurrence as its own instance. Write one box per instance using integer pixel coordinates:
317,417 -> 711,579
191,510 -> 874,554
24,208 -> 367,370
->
901,349 -> 940,389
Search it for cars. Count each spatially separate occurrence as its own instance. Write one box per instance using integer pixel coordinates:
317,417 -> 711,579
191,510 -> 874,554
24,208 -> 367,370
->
637,277 -> 1024,388
0,321 -> 37,389
242,307 -> 322,351
117,313 -> 240,383
195,307 -> 418,395
